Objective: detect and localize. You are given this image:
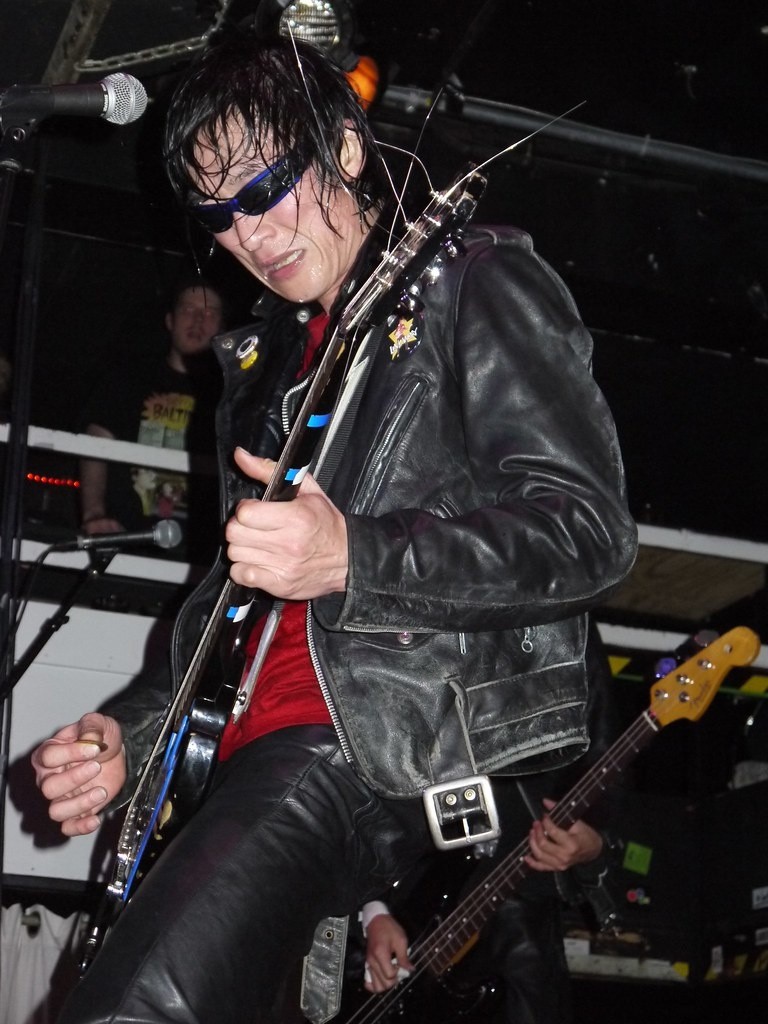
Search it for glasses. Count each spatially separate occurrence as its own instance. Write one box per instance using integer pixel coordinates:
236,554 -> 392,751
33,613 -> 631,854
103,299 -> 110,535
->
194,136 -> 321,233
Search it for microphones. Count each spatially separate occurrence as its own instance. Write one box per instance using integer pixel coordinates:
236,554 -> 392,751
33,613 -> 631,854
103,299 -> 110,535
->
54,520 -> 182,553
0,72 -> 148,125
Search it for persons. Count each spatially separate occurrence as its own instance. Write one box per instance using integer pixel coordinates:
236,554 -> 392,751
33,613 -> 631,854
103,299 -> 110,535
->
31,28 -> 640,1024
338,616 -> 626,1024
75,278 -> 227,557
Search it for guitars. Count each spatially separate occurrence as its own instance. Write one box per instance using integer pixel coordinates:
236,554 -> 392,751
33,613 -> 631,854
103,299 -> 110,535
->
77,166 -> 489,981
342,625 -> 759,1024
